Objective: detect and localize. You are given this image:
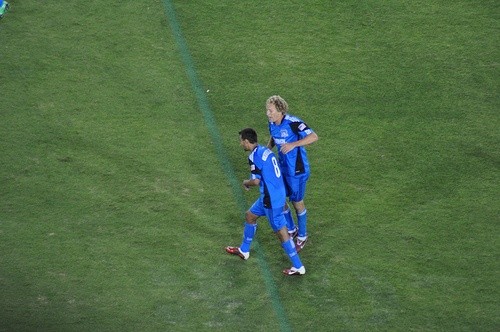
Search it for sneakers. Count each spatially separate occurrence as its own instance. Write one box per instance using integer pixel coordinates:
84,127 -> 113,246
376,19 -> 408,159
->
294,234 -> 309,252
288,226 -> 299,240
225,246 -> 250,260
282,265 -> 306,276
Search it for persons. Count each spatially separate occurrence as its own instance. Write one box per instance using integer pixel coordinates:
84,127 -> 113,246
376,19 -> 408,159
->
224,127 -> 306,276
265,95 -> 319,253
